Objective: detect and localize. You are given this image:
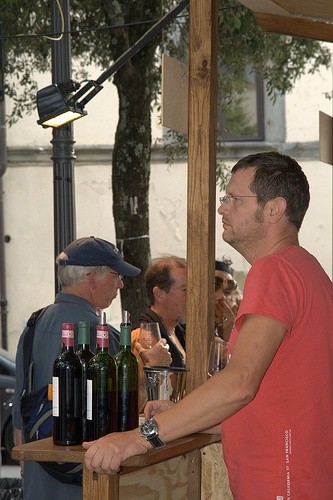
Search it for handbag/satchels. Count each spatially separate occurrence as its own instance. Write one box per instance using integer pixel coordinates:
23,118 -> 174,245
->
20,382 -> 84,484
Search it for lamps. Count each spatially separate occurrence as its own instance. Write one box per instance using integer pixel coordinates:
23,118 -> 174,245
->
35,0 -> 190,129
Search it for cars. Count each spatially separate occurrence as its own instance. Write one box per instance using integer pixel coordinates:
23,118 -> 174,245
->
0,355 -> 19,466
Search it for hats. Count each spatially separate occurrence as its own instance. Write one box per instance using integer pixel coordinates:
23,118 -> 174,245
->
55,236 -> 141,278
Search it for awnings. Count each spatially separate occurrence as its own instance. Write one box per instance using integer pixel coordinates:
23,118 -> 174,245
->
238,2 -> 332,44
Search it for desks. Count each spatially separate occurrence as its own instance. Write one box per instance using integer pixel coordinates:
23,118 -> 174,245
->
12,421 -> 221,499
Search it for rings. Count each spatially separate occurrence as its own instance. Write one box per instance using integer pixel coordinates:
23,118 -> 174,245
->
216,323 -> 222,326
222,318 -> 227,323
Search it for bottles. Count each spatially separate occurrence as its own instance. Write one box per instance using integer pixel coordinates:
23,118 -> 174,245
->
85,324 -> 117,443
113,323 -> 139,433
52,322 -> 84,446
75,321 -> 95,369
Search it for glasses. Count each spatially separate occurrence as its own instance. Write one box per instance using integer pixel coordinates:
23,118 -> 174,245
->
219,194 -> 275,206
214,276 -> 239,292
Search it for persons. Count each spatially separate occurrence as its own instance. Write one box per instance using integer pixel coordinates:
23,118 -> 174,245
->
211,259 -> 241,343
80,151 -> 333,500
9,235 -> 142,499
229,289 -> 242,309
128,254 -> 192,367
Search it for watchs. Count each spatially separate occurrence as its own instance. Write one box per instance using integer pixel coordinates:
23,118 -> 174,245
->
137,418 -> 166,448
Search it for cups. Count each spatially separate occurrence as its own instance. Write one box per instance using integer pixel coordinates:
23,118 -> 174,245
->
140,322 -> 161,349
143,365 -> 190,403
206,341 -> 232,378
